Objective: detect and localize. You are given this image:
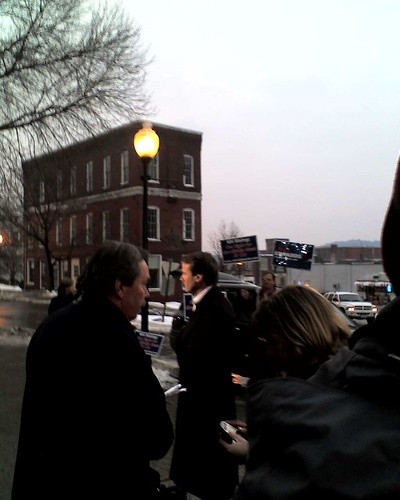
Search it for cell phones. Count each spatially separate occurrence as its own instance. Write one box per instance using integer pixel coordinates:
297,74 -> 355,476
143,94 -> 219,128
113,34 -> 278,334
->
216,420 -> 240,445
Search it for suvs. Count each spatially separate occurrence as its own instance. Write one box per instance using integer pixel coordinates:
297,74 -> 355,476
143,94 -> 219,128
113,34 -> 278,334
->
323,292 -> 377,324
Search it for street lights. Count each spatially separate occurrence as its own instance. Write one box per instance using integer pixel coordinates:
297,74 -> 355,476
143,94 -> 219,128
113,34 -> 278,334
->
134,122 -> 160,332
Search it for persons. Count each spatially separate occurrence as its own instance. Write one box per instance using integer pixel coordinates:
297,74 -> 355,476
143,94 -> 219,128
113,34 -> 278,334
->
12,240 -> 175,500
48,276 -> 80,316
217,160 -> 400,500
169,251 -> 246,500
